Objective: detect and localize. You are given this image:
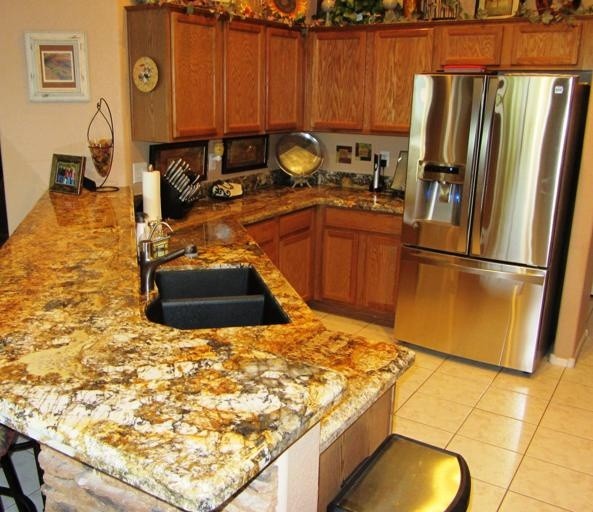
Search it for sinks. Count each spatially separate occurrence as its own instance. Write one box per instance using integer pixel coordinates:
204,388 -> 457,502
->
145,300 -> 290,329
160,263 -> 266,300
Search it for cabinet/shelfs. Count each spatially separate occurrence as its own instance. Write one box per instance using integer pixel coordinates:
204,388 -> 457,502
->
316,199 -> 405,329
261,19 -> 308,136
239,204 -> 317,314
121,1 -> 221,147
368,18 -> 435,140
506,18 -> 593,74
435,15 -> 505,71
303,20 -> 372,136
216,7 -> 267,141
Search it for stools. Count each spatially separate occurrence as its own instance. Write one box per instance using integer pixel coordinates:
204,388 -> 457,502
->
0,424 -> 43,512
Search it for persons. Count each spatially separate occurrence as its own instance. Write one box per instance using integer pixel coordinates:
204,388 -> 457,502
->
57,167 -> 77,184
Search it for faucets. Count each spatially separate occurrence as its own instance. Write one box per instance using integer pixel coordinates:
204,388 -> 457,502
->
139,240 -> 197,293
146,222 -> 173,240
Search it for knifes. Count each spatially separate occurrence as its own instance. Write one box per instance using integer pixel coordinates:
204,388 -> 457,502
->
161,158 -> 202,203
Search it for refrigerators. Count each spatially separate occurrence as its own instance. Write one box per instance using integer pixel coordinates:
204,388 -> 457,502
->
390,71 -> 588,377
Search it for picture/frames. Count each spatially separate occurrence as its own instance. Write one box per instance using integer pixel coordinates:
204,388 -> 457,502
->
22,28 -> 92,105
48,153 -> 85,196
474,0 -> 520,19
221,134 -> 269,176
146,139 -> 209,185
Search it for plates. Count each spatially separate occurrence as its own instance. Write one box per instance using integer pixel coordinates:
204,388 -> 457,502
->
276,133 -> 324,175
132,56 -> 157,95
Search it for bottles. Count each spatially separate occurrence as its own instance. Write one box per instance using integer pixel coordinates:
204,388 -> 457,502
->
148,221 -> 159,242
